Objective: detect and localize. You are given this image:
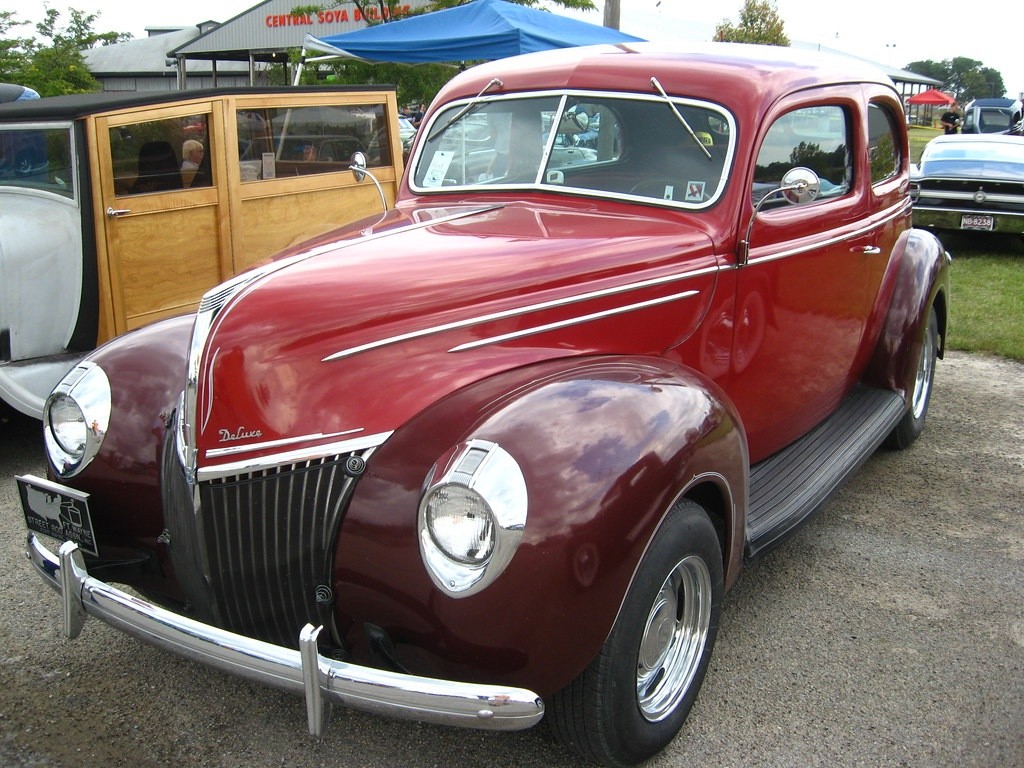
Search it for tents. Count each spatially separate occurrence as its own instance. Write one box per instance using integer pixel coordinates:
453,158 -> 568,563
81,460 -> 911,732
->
292,0 -> 649,90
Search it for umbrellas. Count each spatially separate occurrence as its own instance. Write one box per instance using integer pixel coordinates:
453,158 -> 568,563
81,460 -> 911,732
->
907,89 -> 954,126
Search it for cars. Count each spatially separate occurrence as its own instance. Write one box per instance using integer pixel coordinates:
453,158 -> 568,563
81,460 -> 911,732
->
908,97 -> 1024,242
240,108 -> 600,188
15,38 -> 953,768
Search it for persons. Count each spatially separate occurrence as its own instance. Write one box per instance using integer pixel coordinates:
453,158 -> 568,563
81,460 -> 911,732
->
941,102 -> 961,134
179,140 -> 204,187
401,103 -> 425,127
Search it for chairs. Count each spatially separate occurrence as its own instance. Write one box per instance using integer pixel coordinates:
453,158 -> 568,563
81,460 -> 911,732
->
129,141 -> 184,194
190,143 -> 213,188
